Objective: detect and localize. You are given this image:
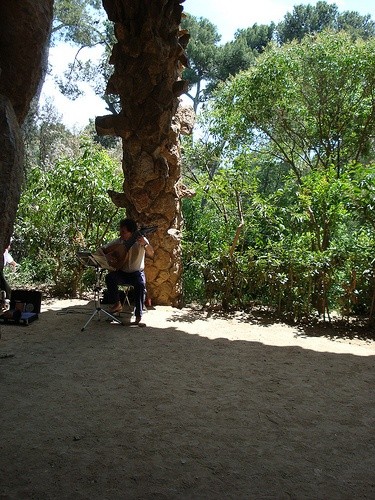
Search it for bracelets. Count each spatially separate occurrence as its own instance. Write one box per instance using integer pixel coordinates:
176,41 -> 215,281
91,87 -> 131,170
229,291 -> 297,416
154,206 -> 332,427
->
143,243 -> 150,249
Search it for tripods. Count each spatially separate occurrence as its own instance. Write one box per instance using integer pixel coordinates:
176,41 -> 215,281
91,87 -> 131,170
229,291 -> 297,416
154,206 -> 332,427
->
75,252 -> 126,332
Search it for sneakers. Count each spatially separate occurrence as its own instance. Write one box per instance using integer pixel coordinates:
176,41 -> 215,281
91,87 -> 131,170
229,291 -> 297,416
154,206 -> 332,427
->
135,317 -> 146,327
106,306 -> 124,313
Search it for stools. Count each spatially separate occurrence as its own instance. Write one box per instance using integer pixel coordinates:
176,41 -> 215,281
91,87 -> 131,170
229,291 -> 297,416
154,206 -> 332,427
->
109,284 -> 138,319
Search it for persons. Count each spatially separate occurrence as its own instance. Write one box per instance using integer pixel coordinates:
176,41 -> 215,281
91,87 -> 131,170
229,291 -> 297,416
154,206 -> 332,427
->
96,218 -> 155,327
